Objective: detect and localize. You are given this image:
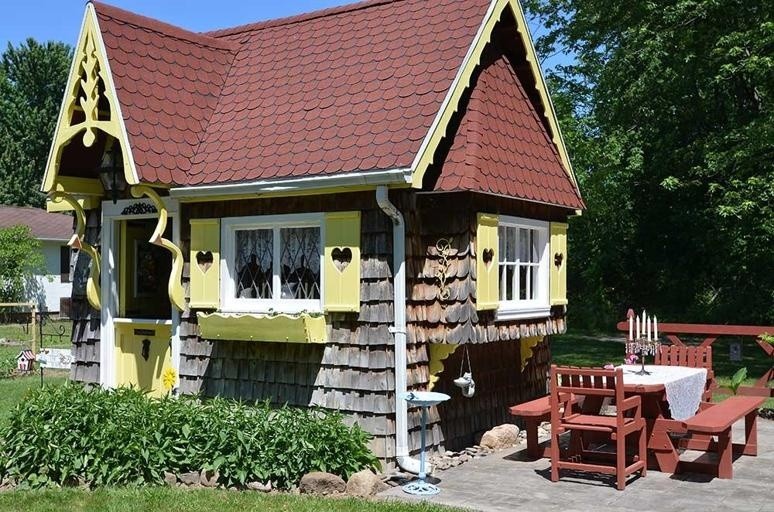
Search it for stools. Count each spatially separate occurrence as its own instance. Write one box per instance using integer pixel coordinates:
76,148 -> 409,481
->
402,392 -> 451,495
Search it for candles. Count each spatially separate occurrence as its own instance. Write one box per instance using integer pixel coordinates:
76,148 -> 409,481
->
629,315 -> 634,340
641,309 -> 646,335
653,314 -> 659,340
647,315 -> 652,341
636,314 -> 640,339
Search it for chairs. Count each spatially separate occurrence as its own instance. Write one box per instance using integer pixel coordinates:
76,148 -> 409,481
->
549,363 -> 647,490
655,344 -> 714,401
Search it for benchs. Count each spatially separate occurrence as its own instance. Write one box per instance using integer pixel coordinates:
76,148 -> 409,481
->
686,395 -> 767,479
509,392 -> 590,459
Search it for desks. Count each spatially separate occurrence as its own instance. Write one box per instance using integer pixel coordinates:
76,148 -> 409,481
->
569,362 -> 708,474
